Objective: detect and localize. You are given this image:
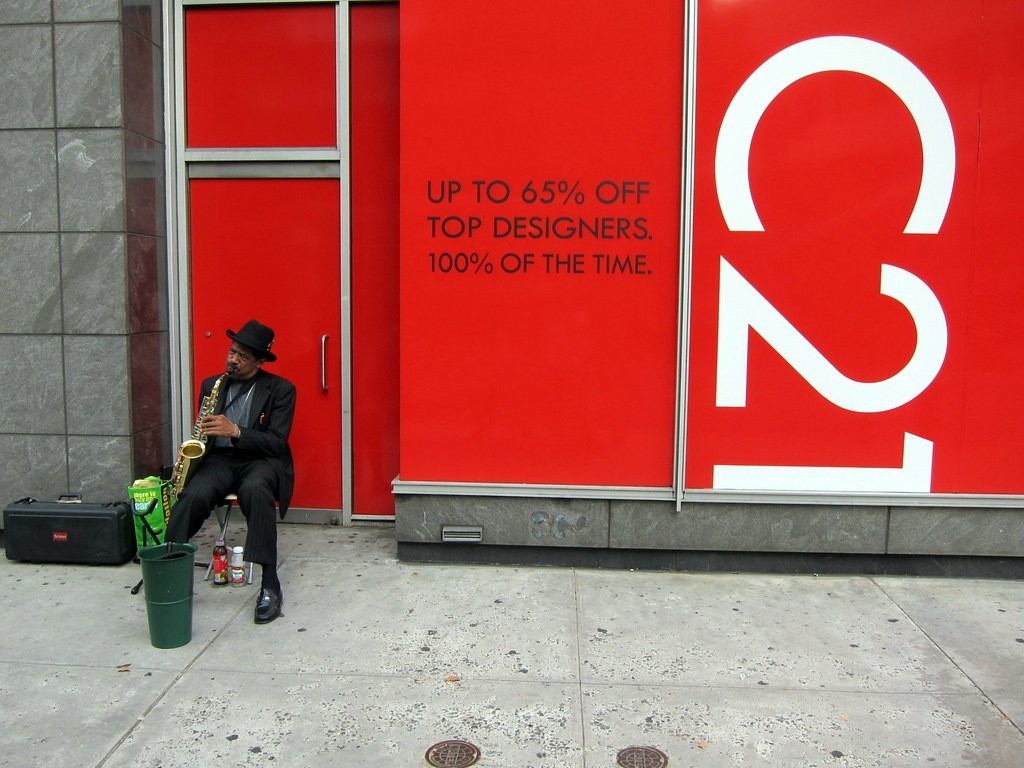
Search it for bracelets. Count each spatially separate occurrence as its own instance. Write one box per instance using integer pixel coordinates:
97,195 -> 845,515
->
235,424 -> 242,439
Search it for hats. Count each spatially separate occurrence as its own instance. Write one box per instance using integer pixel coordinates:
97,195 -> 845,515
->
225,319 -> 276,362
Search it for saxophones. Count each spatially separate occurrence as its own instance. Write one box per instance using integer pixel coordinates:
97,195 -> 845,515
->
168,368 -> 234,500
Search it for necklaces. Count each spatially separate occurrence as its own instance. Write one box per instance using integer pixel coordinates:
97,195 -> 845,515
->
229,382 -> 257,425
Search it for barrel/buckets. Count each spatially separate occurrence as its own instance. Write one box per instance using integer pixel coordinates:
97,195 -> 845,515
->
138,540 -> 198,650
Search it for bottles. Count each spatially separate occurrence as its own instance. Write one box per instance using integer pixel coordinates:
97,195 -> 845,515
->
213,539 -> 229,584
230,547 -> 248,586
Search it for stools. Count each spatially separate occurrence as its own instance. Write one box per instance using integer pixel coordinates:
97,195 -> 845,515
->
193,494 -> 256,583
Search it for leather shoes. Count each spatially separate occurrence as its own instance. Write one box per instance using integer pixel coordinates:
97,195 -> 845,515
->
254,587 -> 282,624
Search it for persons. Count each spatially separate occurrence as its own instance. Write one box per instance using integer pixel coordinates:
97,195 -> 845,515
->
162,319 -> 297,624
